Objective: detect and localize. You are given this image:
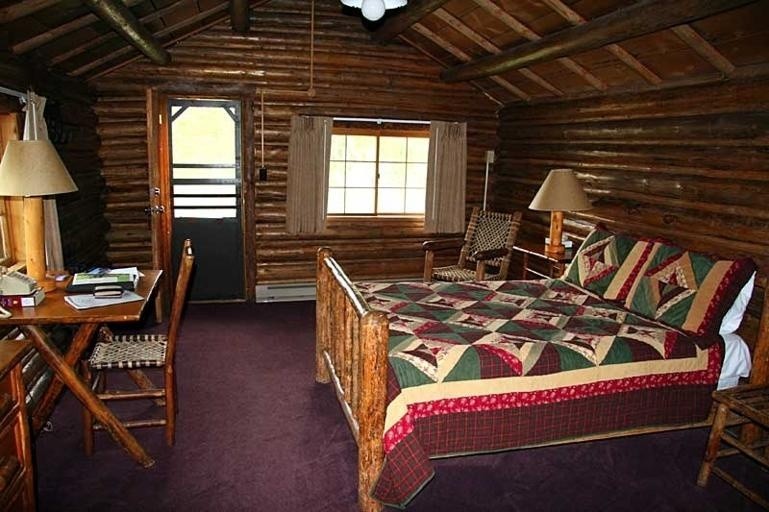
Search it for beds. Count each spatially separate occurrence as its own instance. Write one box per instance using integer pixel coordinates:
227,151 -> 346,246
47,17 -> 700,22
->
315,221 -> 769,512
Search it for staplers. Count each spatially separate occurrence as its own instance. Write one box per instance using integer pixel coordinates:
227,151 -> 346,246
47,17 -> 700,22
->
93,286 -> 123,299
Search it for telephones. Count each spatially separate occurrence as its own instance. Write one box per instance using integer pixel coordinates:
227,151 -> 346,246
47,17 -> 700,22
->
0,265 -> 37,296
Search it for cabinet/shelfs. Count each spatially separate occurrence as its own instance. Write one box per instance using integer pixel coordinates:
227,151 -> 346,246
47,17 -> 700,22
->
0,339 -> 39,512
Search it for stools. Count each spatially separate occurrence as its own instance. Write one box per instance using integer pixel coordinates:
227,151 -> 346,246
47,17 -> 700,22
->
697,382 -> 769,512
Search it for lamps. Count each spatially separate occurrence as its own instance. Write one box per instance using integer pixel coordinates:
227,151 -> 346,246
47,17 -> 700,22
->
341,0 -> 408,22
0,141 -> 82,293
528,169 -> 594,254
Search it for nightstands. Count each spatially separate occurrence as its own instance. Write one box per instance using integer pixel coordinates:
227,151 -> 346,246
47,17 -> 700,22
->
512,242 -> 579,280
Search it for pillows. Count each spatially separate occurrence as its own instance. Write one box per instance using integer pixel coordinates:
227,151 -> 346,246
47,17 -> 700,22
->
624,238 -> 757,343
719,269 -> 757,335
562,220 -> 664,312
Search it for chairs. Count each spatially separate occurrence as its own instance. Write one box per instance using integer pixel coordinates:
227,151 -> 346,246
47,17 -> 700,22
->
420,205 -> 522,282
80,237 -> 194,457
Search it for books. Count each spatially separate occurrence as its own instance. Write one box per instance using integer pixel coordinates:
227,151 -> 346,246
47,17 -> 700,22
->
0,286 -> 46,307
64,267 -> 144,309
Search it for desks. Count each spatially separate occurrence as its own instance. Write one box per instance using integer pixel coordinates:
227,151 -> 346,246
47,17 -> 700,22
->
0,268 -> 163,469
